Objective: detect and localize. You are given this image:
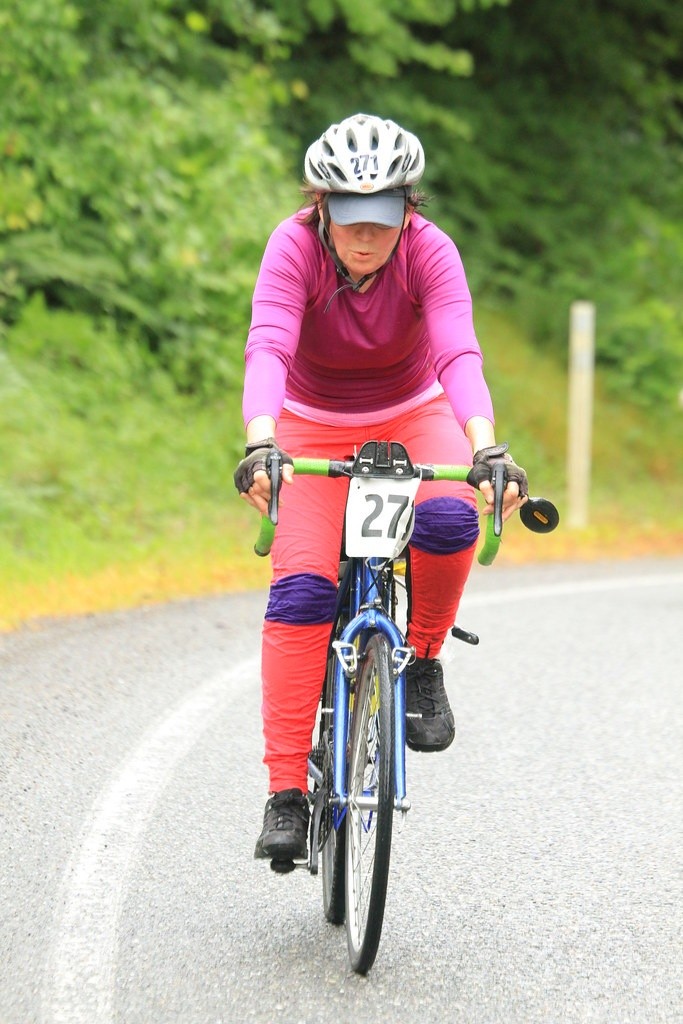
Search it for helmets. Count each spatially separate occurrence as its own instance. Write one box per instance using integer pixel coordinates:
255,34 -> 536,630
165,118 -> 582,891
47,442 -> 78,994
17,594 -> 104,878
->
303,112 -> 426,195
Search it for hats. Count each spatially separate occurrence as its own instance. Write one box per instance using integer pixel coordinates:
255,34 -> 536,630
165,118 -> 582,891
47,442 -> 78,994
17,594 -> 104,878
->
325,185 -> 407,226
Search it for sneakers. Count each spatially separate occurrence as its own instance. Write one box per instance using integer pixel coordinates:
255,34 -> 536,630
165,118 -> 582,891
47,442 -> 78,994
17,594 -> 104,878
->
403,656 -> 455,753
254,791 -> 310,862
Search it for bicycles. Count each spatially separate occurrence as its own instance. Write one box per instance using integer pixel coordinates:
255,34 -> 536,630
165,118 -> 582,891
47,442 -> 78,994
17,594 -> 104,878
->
252,437 -> 560,975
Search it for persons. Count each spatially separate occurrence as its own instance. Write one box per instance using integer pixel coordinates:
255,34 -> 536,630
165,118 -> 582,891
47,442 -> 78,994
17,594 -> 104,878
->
234,112 -> 528,860
